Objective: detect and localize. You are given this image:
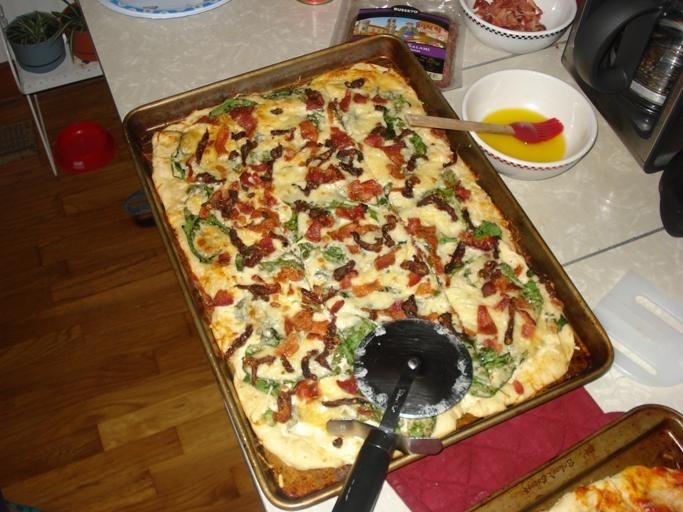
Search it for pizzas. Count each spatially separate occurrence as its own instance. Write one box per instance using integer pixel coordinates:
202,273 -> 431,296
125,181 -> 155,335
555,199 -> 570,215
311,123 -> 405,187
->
144,62 -> 579,497
548,463 -> 682,512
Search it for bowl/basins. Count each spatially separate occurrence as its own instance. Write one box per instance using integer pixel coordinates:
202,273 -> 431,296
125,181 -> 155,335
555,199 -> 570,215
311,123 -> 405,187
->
460,65 -> 600,183
459,1 -> 579,56
51,121 -> 116,174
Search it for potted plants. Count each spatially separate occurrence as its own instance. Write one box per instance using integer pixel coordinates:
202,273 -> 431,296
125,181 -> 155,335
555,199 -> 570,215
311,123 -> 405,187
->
2,10 -> 66,73
50,0 -> 97,62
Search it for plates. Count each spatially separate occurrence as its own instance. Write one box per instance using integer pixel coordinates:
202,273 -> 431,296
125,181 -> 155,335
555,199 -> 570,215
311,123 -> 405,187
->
100,0 -> 233,20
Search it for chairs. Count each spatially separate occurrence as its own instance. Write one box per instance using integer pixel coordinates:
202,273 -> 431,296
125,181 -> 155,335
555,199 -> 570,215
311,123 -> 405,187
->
0,3 -> 104,177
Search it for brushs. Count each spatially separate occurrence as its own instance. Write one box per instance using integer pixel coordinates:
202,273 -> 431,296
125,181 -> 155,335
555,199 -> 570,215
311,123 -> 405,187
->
404,111 -> 564,144
